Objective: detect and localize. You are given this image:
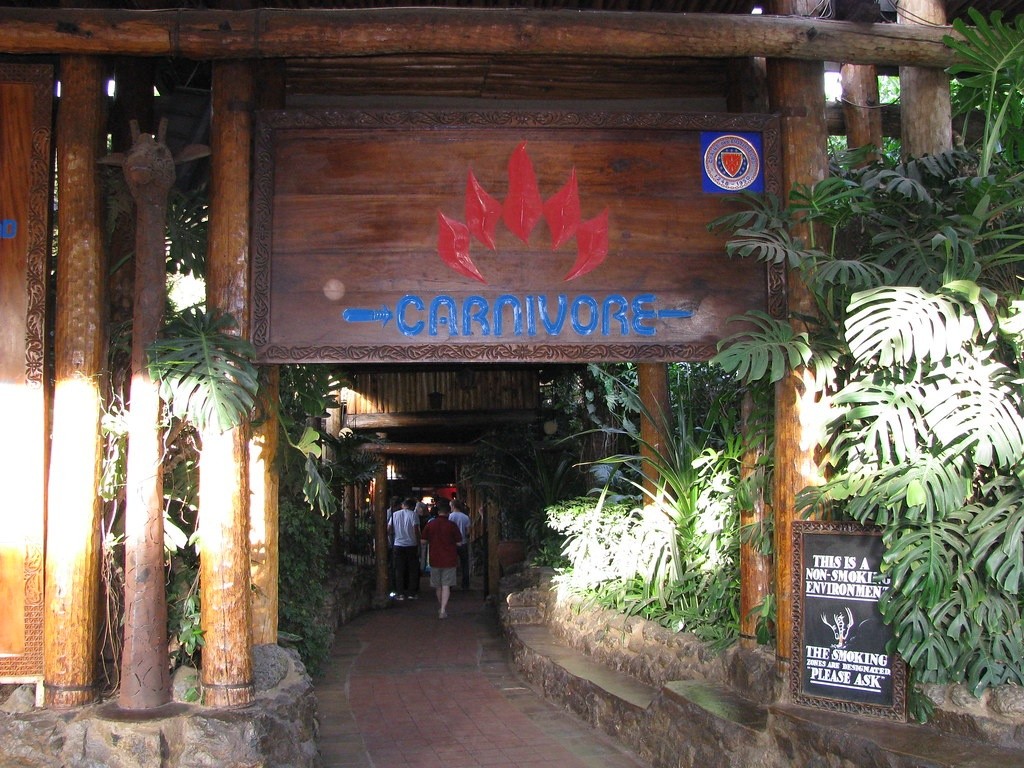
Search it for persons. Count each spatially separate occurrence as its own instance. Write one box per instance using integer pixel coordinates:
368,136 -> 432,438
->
420,500 -> 462,619
362,492 -> 484,599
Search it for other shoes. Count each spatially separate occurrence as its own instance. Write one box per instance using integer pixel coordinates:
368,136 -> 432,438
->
438,608 -> 448,619
396,594 -> 404,600
408,594 -> 419,599
